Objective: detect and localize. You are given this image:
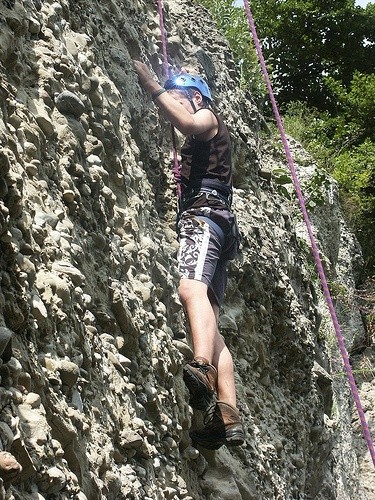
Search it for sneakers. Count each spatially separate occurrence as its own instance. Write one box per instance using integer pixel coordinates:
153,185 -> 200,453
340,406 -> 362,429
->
183,356 -> 217,408
190,402 -> 244,449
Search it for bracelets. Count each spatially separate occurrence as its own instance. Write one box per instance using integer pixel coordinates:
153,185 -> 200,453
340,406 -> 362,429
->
152,88 -> 166,102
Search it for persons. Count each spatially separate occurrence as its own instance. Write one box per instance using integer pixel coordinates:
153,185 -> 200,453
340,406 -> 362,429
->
129,57 -> 246,450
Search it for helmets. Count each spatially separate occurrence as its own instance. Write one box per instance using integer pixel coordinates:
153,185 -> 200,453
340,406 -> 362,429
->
164,73 -> 214,102
